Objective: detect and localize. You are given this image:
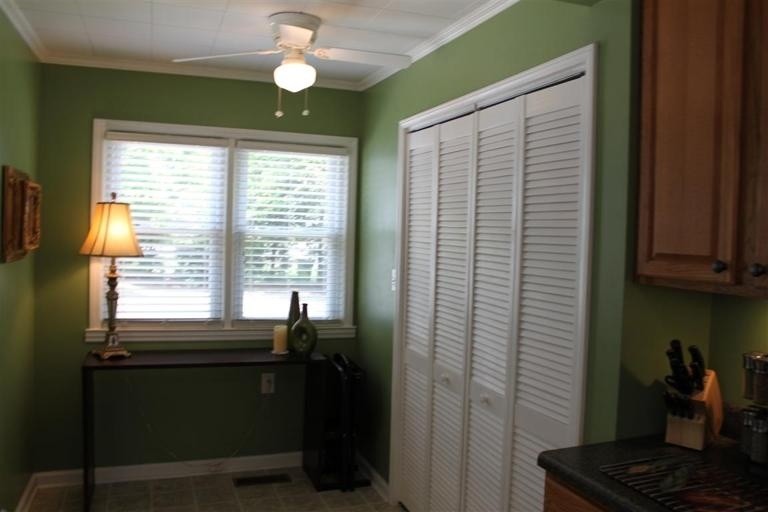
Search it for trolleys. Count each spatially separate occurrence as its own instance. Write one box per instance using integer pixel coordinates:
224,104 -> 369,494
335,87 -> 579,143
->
170,10 -> 412,118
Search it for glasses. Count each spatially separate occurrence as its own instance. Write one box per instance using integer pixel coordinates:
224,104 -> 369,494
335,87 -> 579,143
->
271,58 -> 318,96
80,192 -> 144,359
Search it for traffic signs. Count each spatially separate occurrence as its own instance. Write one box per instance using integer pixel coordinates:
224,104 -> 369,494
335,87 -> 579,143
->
260,371 -> 275,395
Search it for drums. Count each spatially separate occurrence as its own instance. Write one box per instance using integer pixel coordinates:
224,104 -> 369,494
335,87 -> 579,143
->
665,340 -> 705,393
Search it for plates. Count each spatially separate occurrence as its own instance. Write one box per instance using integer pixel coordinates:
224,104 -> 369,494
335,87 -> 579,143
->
638,4 -> 768,297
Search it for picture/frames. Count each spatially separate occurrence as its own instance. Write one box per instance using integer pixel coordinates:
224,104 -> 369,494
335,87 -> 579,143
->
80,349 -> 330,511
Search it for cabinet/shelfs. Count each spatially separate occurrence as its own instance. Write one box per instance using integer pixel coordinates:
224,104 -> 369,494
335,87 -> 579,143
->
739,352 -> 768,463
287,291 -> 316,355
